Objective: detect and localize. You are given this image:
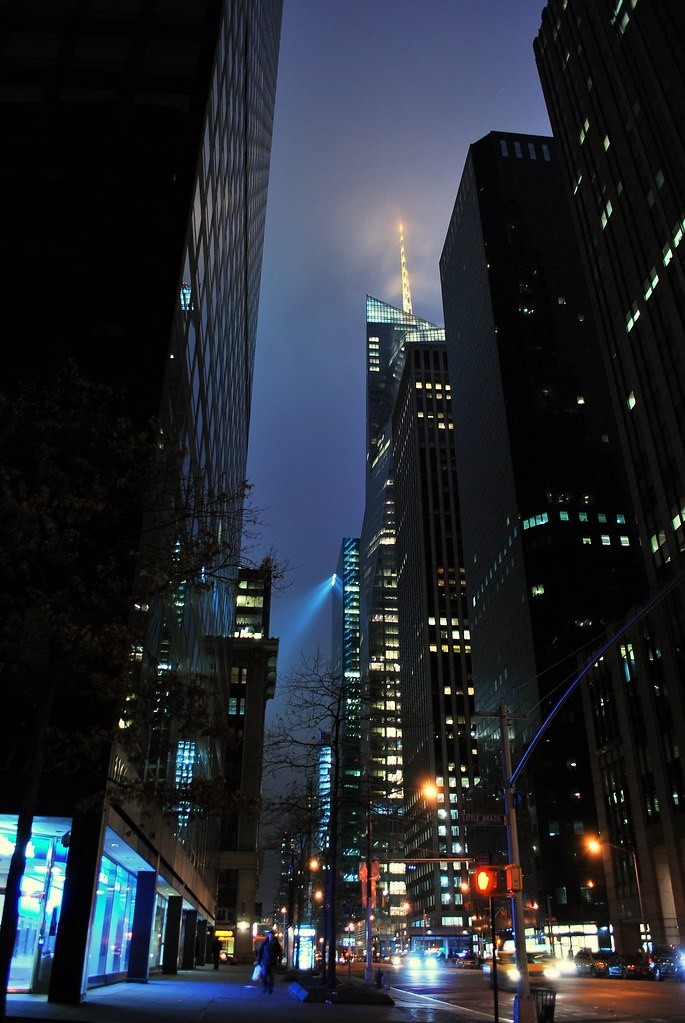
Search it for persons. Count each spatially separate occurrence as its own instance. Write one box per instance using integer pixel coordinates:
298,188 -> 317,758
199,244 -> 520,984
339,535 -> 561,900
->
210,939 -> 222,970
256,931 -> 282,994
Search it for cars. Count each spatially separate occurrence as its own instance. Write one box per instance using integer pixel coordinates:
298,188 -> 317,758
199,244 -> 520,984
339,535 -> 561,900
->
333,937 -> 481,970
572,946 -> 685,981
482,949 -> 560,995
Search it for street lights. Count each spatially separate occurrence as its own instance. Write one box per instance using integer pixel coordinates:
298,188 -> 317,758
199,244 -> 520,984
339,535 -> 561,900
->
585,837 -> 651,955
363,785 -> 439,987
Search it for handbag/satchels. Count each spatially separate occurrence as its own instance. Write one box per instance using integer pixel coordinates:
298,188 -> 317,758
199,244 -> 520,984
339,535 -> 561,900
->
252,965 -> 261,981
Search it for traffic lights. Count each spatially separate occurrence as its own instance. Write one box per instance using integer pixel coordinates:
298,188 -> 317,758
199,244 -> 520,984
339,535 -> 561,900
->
473,864 -> 497,897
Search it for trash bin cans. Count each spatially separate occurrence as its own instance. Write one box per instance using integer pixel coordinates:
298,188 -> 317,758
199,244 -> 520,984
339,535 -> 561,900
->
530,988 -> 557,1023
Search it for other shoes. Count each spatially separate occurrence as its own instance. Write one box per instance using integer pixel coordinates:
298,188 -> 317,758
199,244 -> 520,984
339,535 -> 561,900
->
268,989 -> 273,994
262,984 -> 267,992
